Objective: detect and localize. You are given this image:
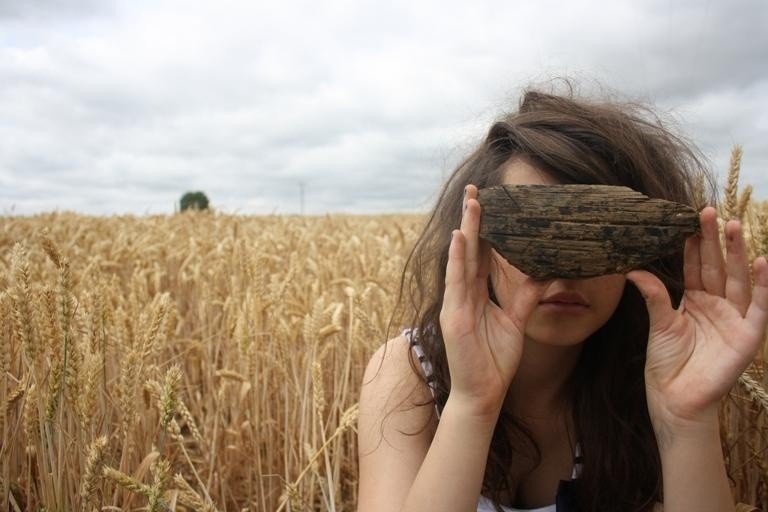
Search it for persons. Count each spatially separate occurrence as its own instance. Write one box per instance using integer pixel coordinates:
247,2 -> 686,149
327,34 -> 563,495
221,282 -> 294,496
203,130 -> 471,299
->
354,90 -> 768,512
